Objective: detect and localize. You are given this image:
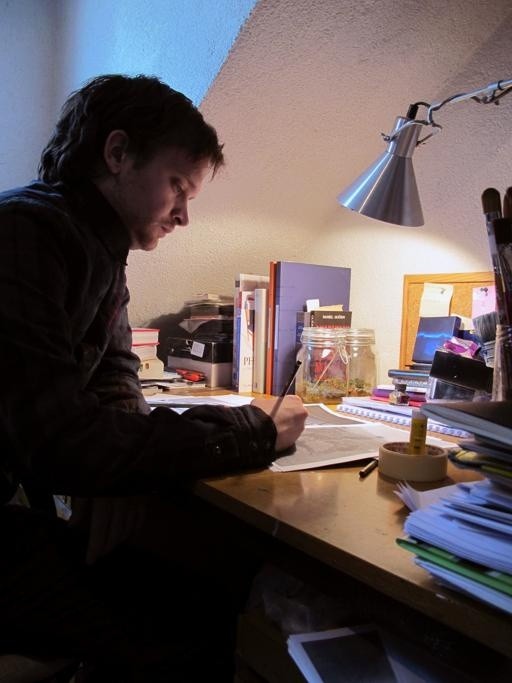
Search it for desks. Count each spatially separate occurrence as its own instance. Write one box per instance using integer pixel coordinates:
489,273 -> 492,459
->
114,387 -> 512,662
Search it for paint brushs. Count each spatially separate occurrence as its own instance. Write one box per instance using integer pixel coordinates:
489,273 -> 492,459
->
482,187 -> 512,326
470,312 -> 504,373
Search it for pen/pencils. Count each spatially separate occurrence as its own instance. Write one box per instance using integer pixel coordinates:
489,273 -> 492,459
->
281,360 -> 302,396
359,456 -> 377,477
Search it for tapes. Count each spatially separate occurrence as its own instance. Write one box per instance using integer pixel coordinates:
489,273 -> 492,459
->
378,440 -> 448,483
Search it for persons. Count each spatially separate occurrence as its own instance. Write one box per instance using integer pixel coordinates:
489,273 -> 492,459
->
1,74 -> 308,683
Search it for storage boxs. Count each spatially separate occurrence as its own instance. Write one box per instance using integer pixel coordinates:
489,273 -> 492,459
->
166,336 -> 233,388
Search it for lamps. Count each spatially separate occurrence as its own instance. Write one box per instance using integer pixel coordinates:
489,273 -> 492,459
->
334,79 -> 512,227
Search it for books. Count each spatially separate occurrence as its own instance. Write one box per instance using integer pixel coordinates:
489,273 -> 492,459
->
394,402 -> 512,616
232,260 -> 351,398
130,329 -> 165,380
411,317 -> 461,363
336,369 -> 476,439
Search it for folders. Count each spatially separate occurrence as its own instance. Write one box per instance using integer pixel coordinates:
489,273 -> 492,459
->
265,261 -> 277,396
273,261 -> 351,397
